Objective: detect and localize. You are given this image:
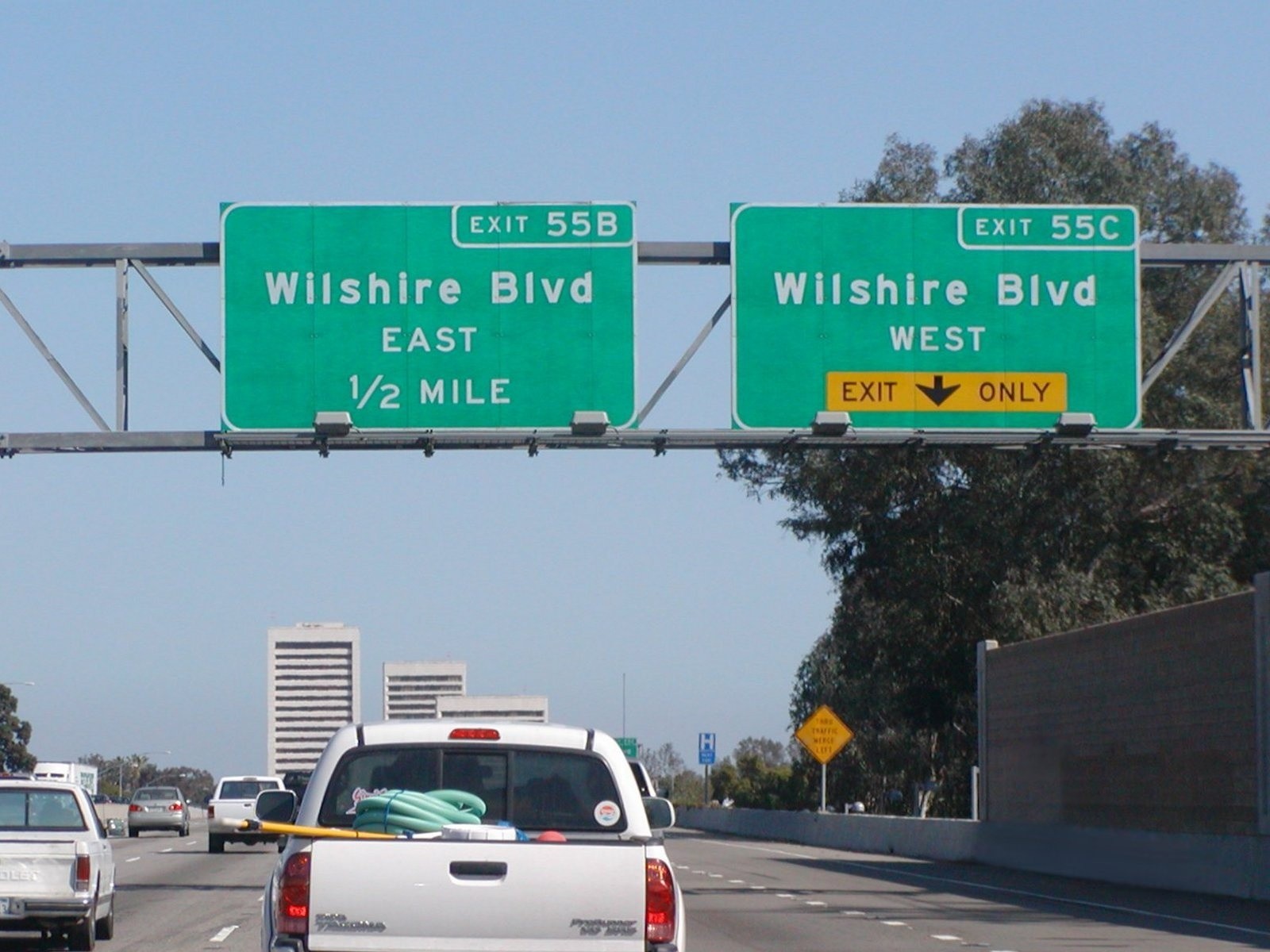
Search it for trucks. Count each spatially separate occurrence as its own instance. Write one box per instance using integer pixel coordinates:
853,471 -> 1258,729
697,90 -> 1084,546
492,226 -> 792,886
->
33,761 -> 99,798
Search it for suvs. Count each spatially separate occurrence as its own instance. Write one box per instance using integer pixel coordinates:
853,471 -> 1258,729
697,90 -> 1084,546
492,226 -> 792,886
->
126,785 -> 191,838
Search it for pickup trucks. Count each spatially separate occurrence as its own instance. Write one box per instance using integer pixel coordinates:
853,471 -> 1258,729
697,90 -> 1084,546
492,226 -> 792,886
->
0,780 -> 126,952
202,775 -> 291,854
253,717 -> 685,952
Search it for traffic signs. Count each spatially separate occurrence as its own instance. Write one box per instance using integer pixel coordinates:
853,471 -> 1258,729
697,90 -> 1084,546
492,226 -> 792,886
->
726,202 -> 1146,436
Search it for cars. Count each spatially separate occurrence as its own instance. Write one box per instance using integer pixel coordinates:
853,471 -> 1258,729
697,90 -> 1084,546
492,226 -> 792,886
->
92,794 -> 112,804
110,796 -> 131,803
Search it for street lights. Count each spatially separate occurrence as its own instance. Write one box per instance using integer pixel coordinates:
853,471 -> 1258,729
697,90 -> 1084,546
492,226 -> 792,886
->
118,748 -> 172,802
